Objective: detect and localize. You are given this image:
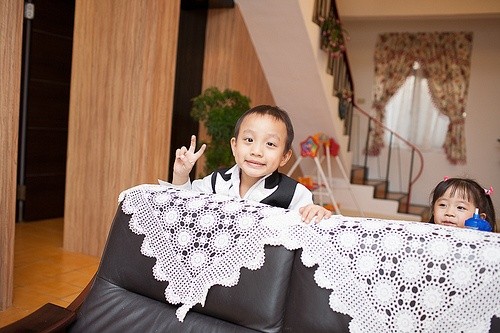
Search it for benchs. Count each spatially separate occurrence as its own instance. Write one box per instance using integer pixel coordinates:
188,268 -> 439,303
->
0,184 -> 500,333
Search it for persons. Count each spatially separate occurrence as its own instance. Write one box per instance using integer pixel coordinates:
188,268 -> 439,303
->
172,105 -> 332,223
428,176 -> 497,232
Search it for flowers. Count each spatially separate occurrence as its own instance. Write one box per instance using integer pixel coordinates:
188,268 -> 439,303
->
321,9 -> 350,58
334,89 -> 354,118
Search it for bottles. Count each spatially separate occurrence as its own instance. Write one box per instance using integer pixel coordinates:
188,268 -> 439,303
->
464,208 -> 493,232
